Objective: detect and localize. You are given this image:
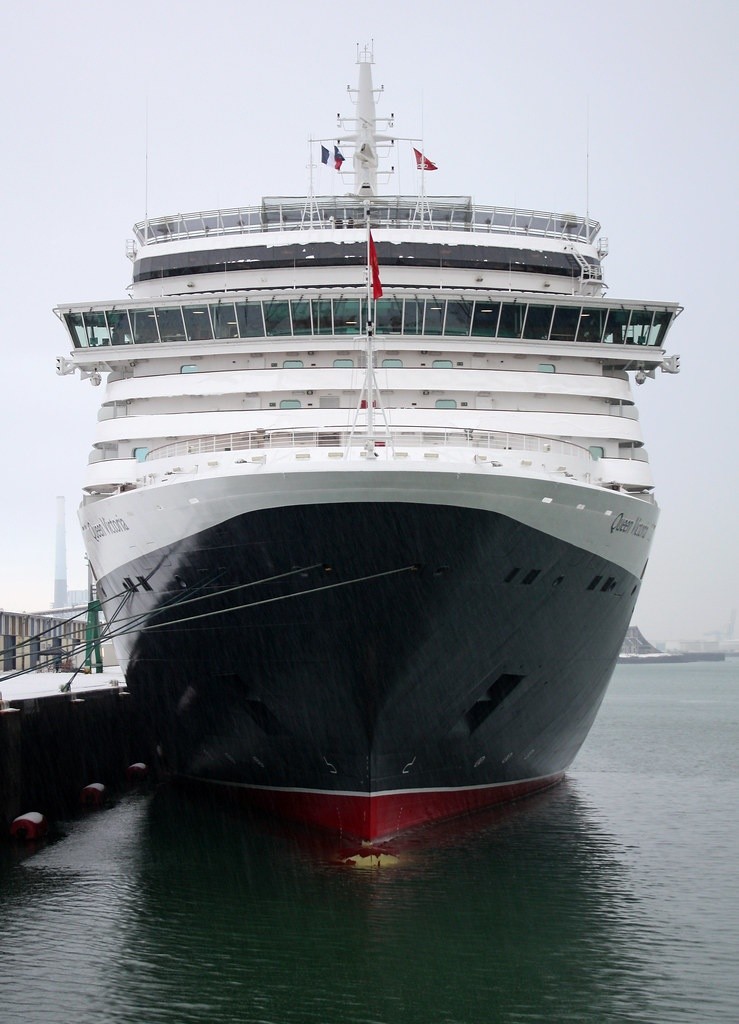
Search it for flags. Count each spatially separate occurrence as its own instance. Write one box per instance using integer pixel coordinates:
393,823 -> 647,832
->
369,233 -> 383,301
321,145 -> 329,164
413,148 -> 438,170
334,147 -> 346,170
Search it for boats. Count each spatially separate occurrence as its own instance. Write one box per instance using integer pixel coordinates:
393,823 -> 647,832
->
56,32 -> 687,840
